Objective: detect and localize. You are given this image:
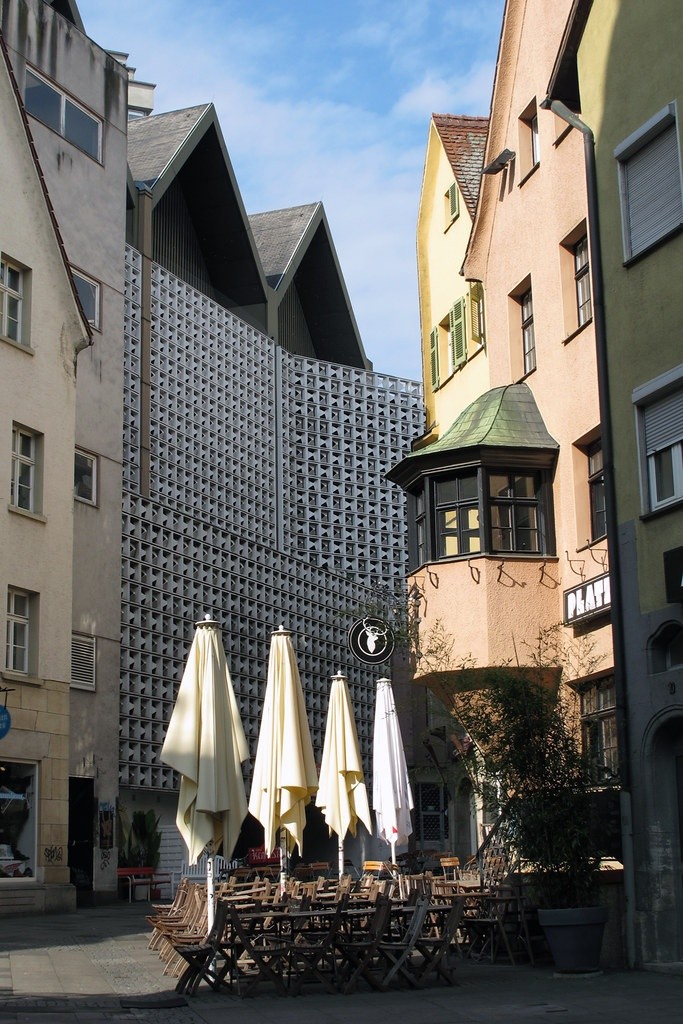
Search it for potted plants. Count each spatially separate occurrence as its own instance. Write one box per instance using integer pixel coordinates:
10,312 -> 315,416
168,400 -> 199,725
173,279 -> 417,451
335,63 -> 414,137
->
334,585 -> 620,975
118,808 -> 163,901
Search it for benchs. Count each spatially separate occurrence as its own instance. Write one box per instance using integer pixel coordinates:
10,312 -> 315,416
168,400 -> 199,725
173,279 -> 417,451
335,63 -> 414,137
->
117,866 -> 171,903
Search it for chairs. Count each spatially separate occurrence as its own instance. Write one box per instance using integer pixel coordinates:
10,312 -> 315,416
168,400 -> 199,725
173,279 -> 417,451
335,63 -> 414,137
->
146,848 -> 552,1000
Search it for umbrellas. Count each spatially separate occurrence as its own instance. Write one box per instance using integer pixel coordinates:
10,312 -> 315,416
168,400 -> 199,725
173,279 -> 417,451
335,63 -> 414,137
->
370,674 -> 416,884
163,612 -> 253,992
316,669 -> 375,886
251,622 -> 320,902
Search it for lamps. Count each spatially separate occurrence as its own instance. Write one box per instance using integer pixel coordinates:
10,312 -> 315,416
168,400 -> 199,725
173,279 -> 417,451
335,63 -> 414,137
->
479,148 -> 515,175
